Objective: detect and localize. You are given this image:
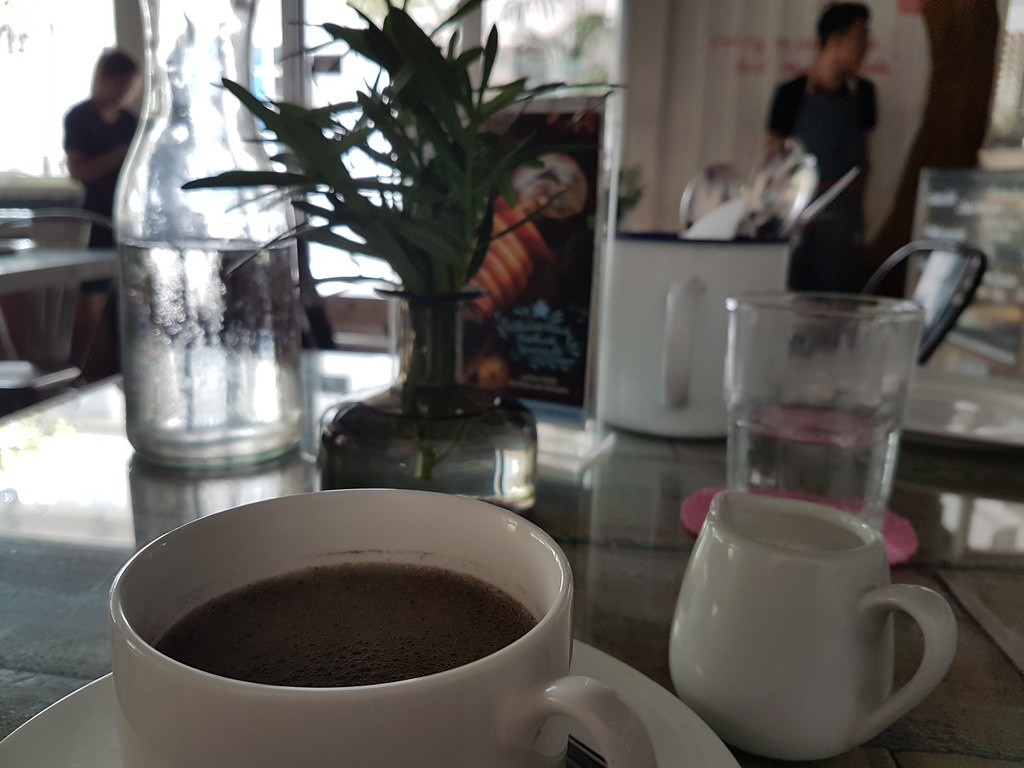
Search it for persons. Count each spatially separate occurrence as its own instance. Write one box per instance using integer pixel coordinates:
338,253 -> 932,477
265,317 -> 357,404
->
767,0 -> 878,355
64,52 -> 139,386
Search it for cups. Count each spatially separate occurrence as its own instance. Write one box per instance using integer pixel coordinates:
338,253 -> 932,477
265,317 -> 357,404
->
594,233 -> 791,441
108,487 -> 657,768
667,492 -> 959,758
728,292 -> 923,536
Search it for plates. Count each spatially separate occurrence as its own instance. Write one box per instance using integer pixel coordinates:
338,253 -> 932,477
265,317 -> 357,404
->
0,636 -> 746,768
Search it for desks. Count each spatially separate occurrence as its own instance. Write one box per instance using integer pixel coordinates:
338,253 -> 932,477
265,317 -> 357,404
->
0,245 -> 124,293
0,349 -> 1024,768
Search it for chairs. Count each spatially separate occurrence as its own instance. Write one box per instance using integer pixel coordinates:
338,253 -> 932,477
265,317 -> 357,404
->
862,239 -> 988,371
0,210 -> 115,417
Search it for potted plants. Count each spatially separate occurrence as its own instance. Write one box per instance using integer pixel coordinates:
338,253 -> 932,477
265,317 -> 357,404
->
176,0 -> 629,513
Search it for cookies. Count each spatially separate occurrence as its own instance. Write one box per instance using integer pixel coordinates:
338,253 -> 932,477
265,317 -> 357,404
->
465,195 -> 549,312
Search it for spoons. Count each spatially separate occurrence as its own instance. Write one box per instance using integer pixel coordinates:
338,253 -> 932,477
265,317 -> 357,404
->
674,140 -> 861,238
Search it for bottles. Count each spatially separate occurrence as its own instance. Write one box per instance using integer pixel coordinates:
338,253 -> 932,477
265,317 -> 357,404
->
112,0 -> 303,476
